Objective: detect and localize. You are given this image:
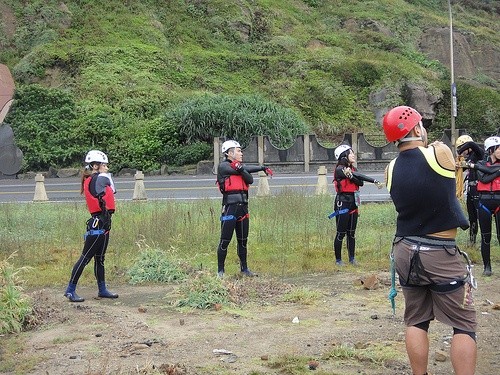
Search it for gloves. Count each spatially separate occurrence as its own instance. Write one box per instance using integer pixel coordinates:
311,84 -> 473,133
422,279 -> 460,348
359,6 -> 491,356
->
342,167 -> 353,180
459,161 -> 472,168
374,179 -> 385,189
263,166 -> 274,177
229,160 -> 244,173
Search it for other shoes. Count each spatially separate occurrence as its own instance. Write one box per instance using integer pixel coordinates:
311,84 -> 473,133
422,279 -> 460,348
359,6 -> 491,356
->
335,259 -> 348,267
240,269 -> 258,277
348,257 -> 357,265
482,266 -> 492,276
217,269 -> 224,279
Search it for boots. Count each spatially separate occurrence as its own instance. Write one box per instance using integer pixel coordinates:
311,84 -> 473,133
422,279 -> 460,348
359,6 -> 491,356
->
98,281 -> 119,298
64,281 -> 84,302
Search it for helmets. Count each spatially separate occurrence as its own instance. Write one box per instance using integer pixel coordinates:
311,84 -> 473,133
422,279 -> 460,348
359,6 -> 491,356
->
85,149 -> 109,164
382,105 -> 423,142
334,145 -> 352,160
455,135 -> 474,148
221,140 -> 241,154
483,136 -> 500,151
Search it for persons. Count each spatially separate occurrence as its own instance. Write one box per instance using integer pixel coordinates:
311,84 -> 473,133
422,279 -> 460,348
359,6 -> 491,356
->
476,136 -> 500,276
64,151 -> 119,303
385,106 -> 477,375
216,140 -> 272,279
333,145 -> 384,267
456,135 -> 484,248
